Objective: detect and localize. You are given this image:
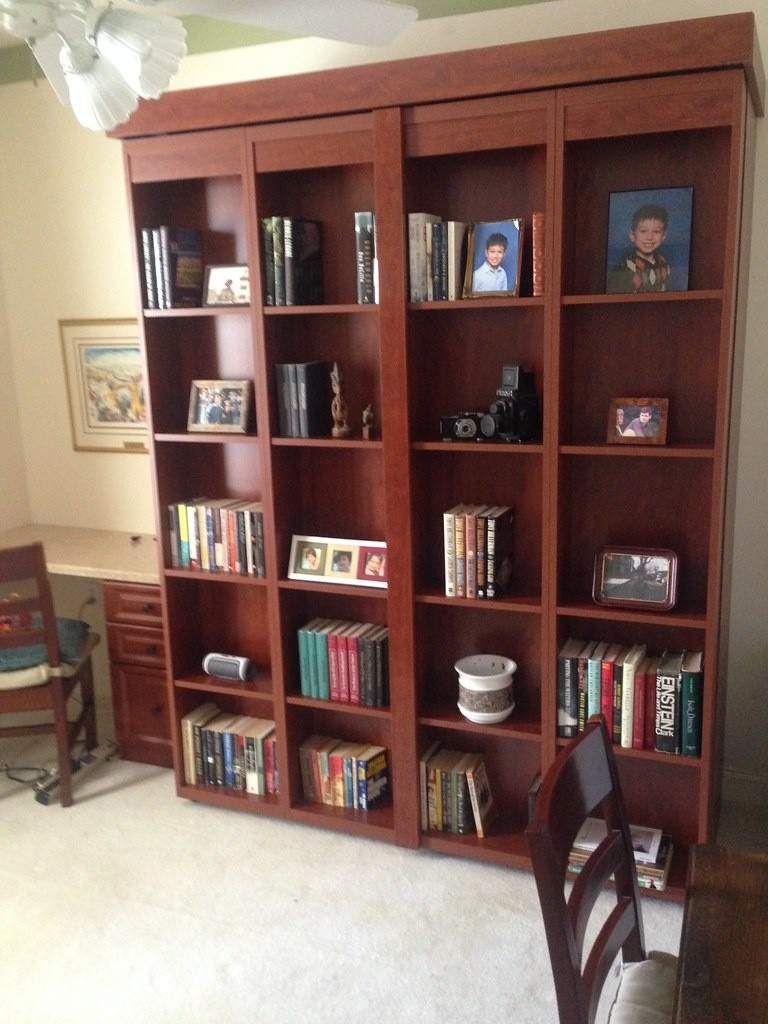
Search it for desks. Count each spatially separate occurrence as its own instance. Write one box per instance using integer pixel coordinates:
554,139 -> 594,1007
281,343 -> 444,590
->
0,523 -> 175,768
674,842 -> 768,1024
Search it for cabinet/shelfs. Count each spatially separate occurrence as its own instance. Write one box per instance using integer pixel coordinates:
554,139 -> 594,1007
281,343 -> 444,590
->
122,10 -> 768,898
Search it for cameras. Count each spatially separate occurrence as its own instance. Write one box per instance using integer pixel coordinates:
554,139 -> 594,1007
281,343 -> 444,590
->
440,413 -> 487,442
480,365 -> 539,444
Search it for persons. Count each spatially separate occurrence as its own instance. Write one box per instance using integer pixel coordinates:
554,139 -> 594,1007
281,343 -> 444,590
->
607,205 -> 670,293
204,393 -> 232,424
218,279 -> 237,304
364,554 -> 384,577
471,233 -> 508,292
616,407 -> 658,437
304,547 -> 320,570
333,551 -> 351,571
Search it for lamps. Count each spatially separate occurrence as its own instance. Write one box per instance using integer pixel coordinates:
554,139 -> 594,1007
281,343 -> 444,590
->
55,0 -> 188,131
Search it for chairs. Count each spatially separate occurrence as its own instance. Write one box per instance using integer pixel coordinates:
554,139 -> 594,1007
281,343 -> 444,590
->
529,712 -> 676,1024
1,541 -> 99,807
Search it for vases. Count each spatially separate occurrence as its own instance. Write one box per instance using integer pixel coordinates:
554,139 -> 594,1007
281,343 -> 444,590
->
453,655 -> 519,724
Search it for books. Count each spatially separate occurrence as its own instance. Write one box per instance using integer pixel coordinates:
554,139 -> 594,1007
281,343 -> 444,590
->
141,212 -> 545,309
443,503 -> 515,599
298,618 -> 387,709
567,817 -> 676,890
420,742 -> 498,838
275,360 -> 330,438
169,496 -> 265,575
181,704 -> 390,811
555,637 -> 704,758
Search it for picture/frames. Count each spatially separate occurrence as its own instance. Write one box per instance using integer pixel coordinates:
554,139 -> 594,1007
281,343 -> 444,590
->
202,264 -> 250,307
606,398 -> 669,445
592,546 -> 679,610
58,318 -> 148,454
462,219 -> 525,297
288,534 -> 388,591
187,379 -> 251,434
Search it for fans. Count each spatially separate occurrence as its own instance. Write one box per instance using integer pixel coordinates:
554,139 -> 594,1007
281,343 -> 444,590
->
0,0 -> 417,105
